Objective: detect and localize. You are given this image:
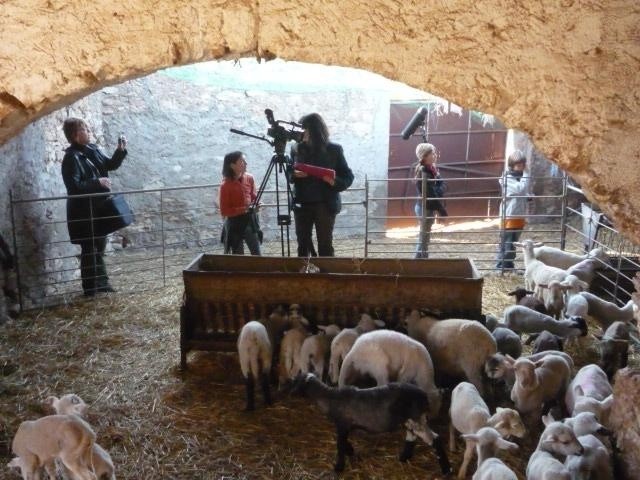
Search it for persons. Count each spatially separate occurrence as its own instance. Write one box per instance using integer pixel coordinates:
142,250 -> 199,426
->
496,151 -> 536,272
413,141 -> 448,259
217,150 -> 266,256
60,117 -> 130,298
283,113 -> 356,257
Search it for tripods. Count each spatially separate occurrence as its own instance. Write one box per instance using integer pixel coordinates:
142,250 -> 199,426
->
240,162 -> 301,257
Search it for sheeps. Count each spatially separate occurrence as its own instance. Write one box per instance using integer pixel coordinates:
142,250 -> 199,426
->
6,413 -> 97,480
45,394 -> 116,480
236,237 -> 640,480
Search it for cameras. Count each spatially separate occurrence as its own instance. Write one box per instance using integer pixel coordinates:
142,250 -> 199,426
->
118,135 -> 123,145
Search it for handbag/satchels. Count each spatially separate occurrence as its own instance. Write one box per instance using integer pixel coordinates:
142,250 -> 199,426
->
100,196 -> 131,233
